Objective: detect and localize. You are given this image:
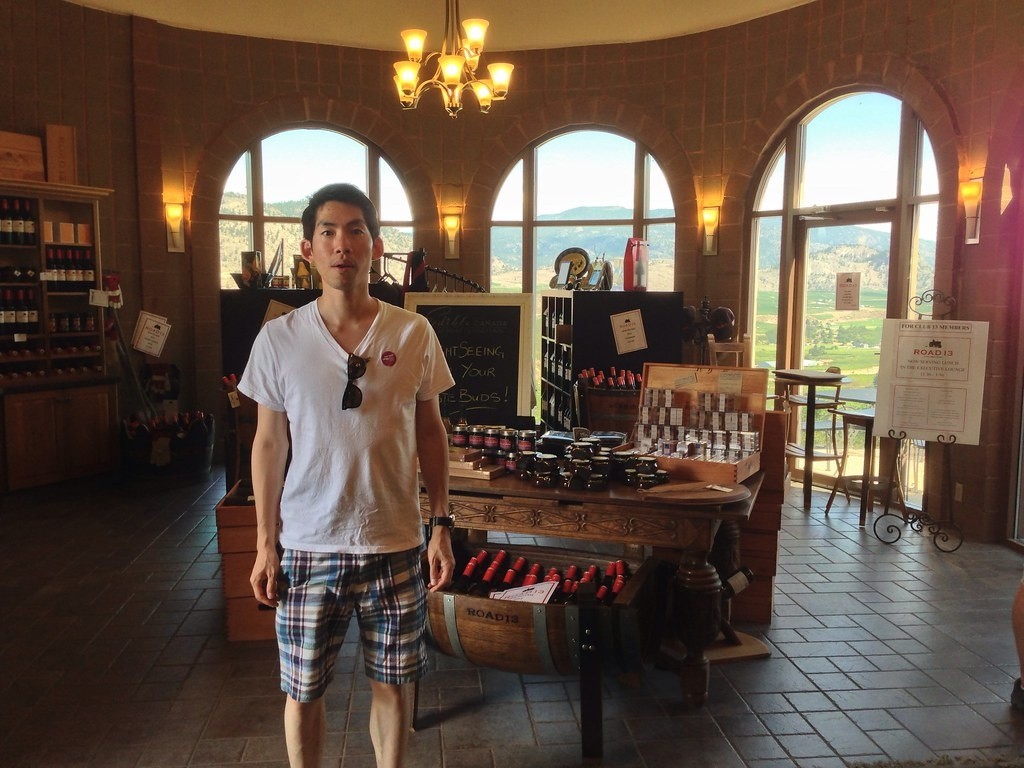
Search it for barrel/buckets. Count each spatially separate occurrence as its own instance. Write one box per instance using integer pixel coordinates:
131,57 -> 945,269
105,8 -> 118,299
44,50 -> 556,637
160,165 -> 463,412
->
120,414 -> 216,489
584,388 -> 641,437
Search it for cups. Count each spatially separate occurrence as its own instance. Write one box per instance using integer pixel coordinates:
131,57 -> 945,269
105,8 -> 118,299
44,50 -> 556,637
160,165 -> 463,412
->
515,438 -> 669,493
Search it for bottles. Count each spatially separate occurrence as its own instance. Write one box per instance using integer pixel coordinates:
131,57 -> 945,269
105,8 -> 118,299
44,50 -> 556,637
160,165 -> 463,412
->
722,567 -> 755,599
542,312 -> 571,431
1,197 -> 104,379
577,366 -> 642,389
450,546 -> 625,605
126,410 -> 208,437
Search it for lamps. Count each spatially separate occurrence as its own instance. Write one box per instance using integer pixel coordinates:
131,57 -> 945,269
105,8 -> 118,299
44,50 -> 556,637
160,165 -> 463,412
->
164,202 -> 185,253
443,213 -> 460,259
959,177 -> 983,245
393,0 -> 515,120
703,206 -> 720,256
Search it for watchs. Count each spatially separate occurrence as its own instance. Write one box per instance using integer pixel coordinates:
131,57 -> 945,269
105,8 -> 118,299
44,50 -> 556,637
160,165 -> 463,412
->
429,514 -> 456,530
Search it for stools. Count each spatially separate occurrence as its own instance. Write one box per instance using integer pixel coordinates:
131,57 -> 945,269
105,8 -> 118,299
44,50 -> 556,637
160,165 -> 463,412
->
800,418 -> 847,470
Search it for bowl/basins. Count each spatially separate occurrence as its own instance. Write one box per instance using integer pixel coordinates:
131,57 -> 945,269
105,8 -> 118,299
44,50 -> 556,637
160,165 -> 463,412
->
233,273 -> 272,289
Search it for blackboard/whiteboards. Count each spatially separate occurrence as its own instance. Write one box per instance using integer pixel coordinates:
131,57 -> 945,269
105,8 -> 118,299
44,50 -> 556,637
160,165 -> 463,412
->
404,292 -> 533,428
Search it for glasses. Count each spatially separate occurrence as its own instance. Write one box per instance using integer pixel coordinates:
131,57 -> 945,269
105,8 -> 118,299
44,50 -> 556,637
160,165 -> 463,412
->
341,353 -> 371,410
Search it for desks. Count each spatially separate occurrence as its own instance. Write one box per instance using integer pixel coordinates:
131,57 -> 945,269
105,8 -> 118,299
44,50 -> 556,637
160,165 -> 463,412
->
418,469 -> 766,760
771,374 -> 853,512
825,407 -> 908,527
816,386 -> 876,408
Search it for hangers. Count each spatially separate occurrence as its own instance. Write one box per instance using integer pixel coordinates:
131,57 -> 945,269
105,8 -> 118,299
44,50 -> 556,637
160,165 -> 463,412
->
375,257 -> 399,284
425,266 -> 449,293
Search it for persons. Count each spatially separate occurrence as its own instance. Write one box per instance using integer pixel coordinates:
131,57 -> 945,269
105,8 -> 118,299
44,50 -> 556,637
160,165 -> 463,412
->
237,183 -> 456,768
1010,576 -> 1024,711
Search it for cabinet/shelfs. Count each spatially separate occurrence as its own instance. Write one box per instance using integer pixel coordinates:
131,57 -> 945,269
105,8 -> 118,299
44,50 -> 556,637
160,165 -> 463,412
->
0,177 -> 123,386
2,383 -> 122,493
540,289 -> 684,432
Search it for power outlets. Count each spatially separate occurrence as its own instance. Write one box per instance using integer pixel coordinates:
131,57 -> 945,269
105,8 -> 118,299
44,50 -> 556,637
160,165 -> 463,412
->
953,481 -> 964,503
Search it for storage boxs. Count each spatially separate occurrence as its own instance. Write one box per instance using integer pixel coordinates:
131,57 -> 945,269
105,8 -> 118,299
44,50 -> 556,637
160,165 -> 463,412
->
213,477 -> 277,643
612,362 -> 769,485
45,124 -> 78,185
0,130 -> 45,181
